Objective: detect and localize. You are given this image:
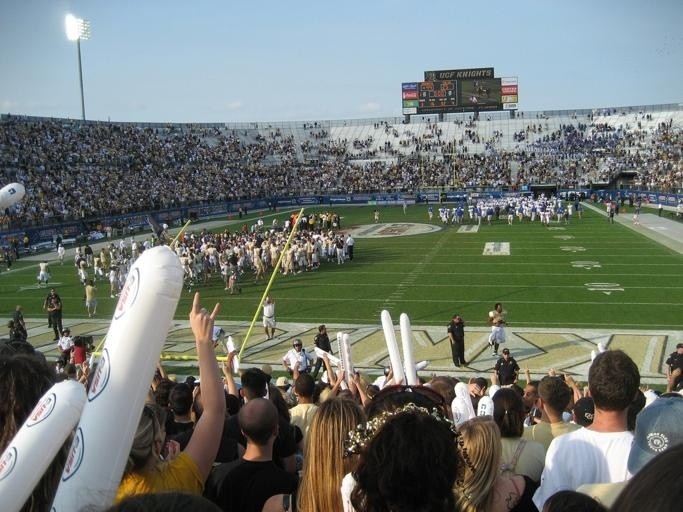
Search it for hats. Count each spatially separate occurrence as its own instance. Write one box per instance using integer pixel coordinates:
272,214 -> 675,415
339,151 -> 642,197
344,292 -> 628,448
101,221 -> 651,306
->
502,348 -> 509,354
275,376 -> 289,387
574,397 -> 593,425
321,370 -> 336,384
627,396 -> 683,477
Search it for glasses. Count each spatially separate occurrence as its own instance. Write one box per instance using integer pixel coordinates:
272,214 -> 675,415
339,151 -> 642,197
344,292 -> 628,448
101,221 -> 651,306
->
146,404 -> 163,442
366,384 -> 448,420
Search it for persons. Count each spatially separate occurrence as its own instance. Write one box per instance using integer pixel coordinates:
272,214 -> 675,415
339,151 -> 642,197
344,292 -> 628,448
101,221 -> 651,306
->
468,78 -> 490,109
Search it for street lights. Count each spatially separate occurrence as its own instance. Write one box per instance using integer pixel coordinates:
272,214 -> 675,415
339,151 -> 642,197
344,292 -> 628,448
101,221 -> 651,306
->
69,16 -> 90,121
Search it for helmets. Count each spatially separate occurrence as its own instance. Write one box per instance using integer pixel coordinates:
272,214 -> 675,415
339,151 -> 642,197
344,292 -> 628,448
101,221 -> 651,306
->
61,327 -> 70,336
292,339 -> 301,348
